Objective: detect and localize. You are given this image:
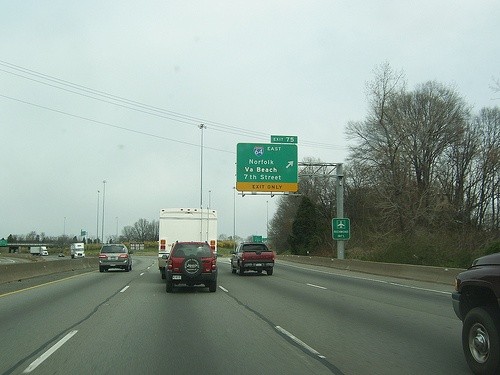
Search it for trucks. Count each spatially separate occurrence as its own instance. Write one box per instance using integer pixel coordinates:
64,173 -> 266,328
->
157,208 -> 218,279
70,242 -> 85,259
29,246 -> 48,256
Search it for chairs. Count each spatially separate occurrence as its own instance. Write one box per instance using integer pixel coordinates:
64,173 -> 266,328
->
196,248 -> 206,256
177,249 -> 185,256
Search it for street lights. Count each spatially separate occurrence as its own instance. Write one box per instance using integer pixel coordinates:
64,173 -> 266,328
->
232,187 -> 236,249
209,190 -> 211,207
198,123 -> 206,207
97,190 -> 100,245
266,200 -> 269,236
102,180 -> 106,245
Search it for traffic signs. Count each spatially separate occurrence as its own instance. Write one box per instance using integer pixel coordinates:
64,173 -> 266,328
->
236,142 -> 298,192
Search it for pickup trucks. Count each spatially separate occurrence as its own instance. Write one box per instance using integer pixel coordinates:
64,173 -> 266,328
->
230,242 -> 275,276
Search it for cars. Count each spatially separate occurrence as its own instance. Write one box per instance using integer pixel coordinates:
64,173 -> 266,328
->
451,252 -> 500,375
59,253 -> 65,257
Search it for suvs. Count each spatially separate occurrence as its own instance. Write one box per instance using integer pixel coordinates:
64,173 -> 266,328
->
162,240 -> 218,293
98,243 -> 132,272
